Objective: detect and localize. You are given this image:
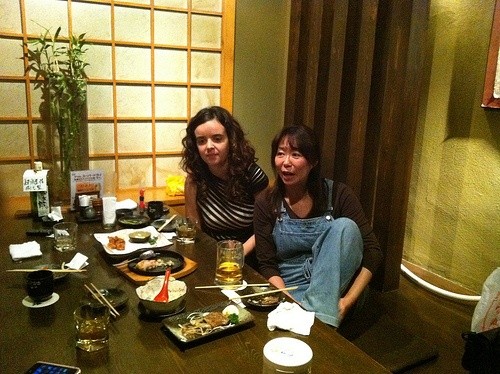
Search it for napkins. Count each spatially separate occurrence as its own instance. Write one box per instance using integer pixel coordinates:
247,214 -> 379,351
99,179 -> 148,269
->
48,206 -> 63,221
266,301 -> 316,336
9,240 -> 42,261
66,252 -> 90,271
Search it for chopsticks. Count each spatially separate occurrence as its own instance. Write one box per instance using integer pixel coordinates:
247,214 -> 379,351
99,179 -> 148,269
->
84,283 -> 120,318
158,214 -> 176,230
6,270 -> 86,272
195,283 -> 298,300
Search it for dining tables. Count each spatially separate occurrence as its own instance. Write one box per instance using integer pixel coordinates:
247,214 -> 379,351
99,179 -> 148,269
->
0,202 -> 391,374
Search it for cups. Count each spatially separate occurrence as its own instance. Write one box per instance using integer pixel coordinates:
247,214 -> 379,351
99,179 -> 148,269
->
215,241 -> 244,289
53,222 -> 78,252
92,198 -> 103,215
25,270 -> 53,301
175,217 -> 198,245
73,302 -> 110,351
147,201 -> 163,219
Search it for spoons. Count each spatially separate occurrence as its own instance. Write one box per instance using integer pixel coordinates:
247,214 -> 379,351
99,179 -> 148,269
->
152,268 -> 171,303
113,250 -> 154,266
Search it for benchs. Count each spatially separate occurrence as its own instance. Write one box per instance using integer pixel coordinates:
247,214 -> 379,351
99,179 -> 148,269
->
336,309 -> 440,374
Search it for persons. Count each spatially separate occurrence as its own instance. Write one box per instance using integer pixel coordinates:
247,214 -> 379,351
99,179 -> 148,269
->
254,123 -> 382,335
180,106 -> 269,269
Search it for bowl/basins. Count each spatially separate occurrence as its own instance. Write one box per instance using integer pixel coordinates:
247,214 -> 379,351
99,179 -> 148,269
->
153,219 -> 176,230
135,286 -> 189,313
117,209 -> 133,221
242,286 -> 288,309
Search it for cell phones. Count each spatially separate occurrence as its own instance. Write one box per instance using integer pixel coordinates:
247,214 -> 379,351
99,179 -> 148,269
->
25,360 -> 82,374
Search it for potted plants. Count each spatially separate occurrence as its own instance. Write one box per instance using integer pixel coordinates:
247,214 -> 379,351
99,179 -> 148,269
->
17,17 -> 91,206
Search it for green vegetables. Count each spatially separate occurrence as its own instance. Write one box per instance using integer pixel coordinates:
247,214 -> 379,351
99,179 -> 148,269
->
227,313 -> 239,324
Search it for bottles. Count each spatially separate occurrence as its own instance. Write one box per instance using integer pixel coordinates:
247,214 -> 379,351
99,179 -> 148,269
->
32,161 -> 51,223
261,337 -> 313,374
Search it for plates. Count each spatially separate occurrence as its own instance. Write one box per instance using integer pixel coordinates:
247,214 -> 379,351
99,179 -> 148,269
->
33,264 -> 70,281
118,215 -> 151,229
129,249 -> 184,276
88,288 -> 126,309
93,225 -> 174,257
162,300 -> 255,344
